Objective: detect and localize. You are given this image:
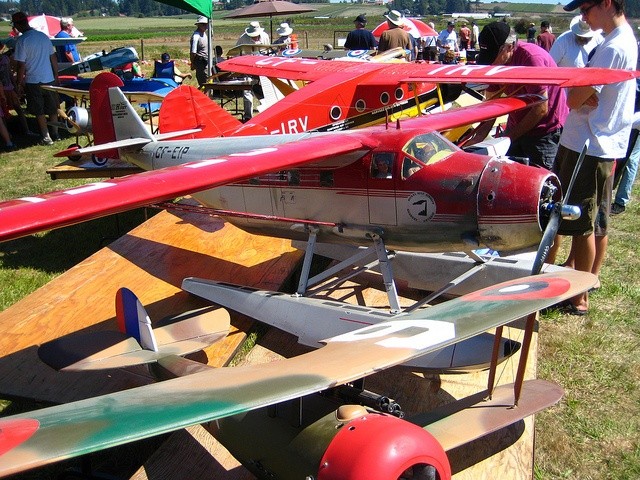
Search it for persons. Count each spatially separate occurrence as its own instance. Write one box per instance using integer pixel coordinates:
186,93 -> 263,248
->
152,53 -> 192,85
10,12 -> 60,146
416,37 -> 424,60
548,15 -> 605,68
0,82 -> 14,151
0,52 -> 36,137
470,22 -> 480,48
437,22 -> 460,61
408,33 -> 418,60
550,0 -> 638,316
526,23 -> 536,42
236,21 -> 271,45
189,14 -> 209,86
213,45 -> 228,64
609,24 -> 639,217
423,22 -> 439,61
378,10 -> 412,61
273,22 -> 294,50
12,26 -> 23,36
344,15 -> 378,50
459,23 -> 471,50
372,156 -> 394,179
54,18 -> 81,112
460,22 -> 569,168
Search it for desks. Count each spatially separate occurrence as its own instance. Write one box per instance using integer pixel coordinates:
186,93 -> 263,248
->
202,79 -> 259,121
47,156 -> 144,252
0,210 -> 305,403
130,249 -> 541,480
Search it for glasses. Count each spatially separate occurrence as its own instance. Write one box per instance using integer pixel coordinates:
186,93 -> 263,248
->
580,3 -> 599,16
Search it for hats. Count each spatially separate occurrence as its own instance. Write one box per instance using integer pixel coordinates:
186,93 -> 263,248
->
322,43 -> 333,51
276,22 -> 293,36
245,22 -> 265,37
162,52 -> 170,61
563,0 -> 589,11
476,22 -> 510,65
570,15 -> 603,38
447,21 -> 455,26
384,10 -> 403,25
194,15 -> 208,26
353,15 -> 367,22
10,12 -> 27,24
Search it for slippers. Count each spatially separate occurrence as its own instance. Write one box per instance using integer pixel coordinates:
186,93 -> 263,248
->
540,299 -> 589,316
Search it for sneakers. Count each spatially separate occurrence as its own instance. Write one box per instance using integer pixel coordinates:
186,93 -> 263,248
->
610,202 -> 625,216
23,130 -> 40,136
7,142 -> 20,151
32,136 -> 53,145
50,132 -> 61,141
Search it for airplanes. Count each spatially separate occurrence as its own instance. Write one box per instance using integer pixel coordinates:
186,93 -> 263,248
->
1,270 -> 599,480
39,84 -> 164,165
160,55 -> 640,177
1,71 -> 590,371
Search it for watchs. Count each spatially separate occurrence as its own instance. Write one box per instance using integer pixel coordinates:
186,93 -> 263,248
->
441,45 -> 444,48
536,21 -> 555,53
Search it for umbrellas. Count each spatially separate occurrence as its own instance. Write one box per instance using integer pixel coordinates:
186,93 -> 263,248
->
10,12 -> 84,39
372,18 -> 439,37
220,1 -> 320,44
